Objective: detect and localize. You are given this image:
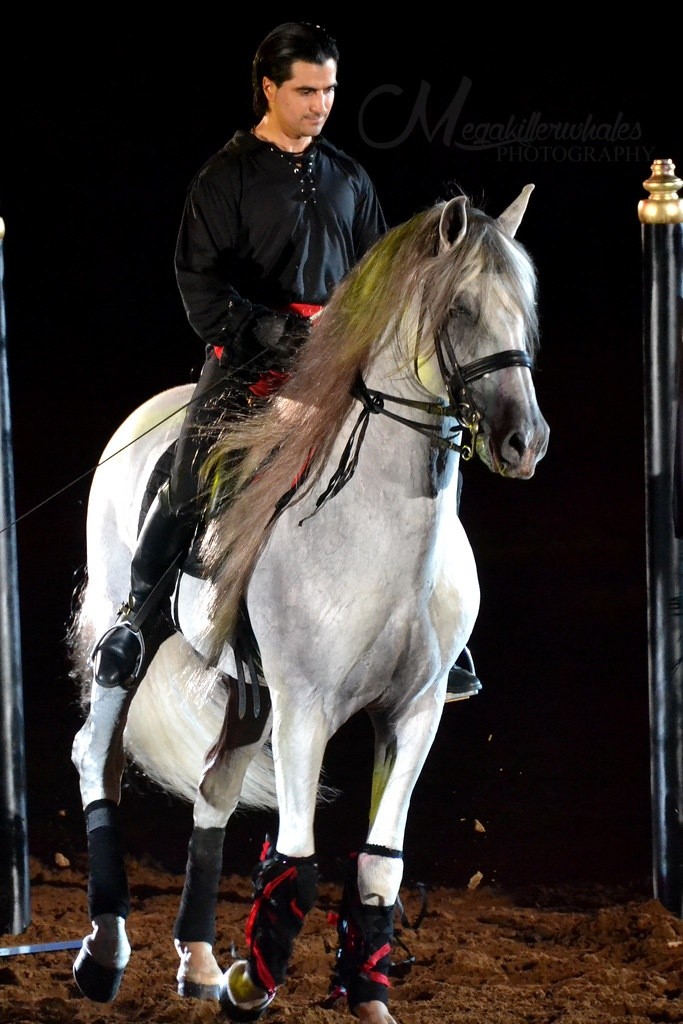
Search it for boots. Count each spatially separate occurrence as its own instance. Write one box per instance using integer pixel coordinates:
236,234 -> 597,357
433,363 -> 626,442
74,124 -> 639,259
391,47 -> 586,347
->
447,662 -> 484,696
93,475 -> 201,688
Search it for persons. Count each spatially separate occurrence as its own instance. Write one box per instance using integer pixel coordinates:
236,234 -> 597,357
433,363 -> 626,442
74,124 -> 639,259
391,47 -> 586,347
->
87,18 -> 392,692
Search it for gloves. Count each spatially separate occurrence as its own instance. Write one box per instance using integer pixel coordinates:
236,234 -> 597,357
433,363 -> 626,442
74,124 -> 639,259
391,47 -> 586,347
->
235,300 -> 310,367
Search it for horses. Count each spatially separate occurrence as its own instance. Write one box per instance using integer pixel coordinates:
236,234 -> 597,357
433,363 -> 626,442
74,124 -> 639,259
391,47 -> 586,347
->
62,185 -> 552,1016
